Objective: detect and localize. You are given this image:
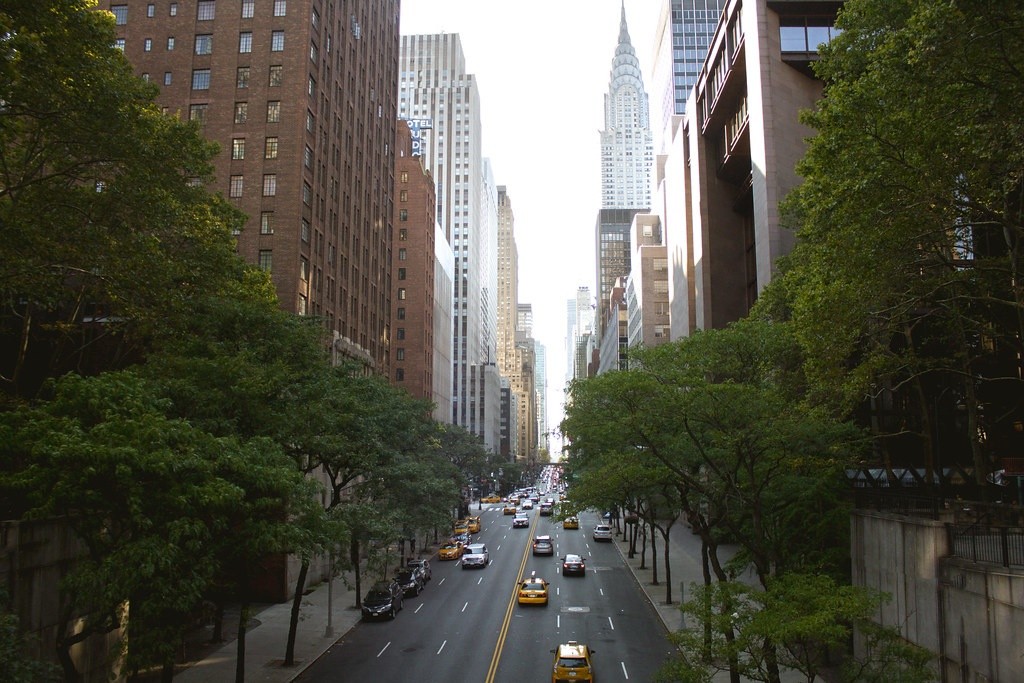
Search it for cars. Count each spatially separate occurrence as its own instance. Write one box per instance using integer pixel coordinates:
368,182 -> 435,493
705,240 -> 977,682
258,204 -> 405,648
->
560,553 -> 586,577
392,559 -> 432,597
462,543 -> 489,569
438,516 -> 481,561
549,640 -> 596,683
593,525 -> 612,541
480,495 -> 501,503
504,486 -> 578,529
362,581 -> 404,621
518,578 -> 550,606
539,465 -> 568,486
532,535 -> 554,556
